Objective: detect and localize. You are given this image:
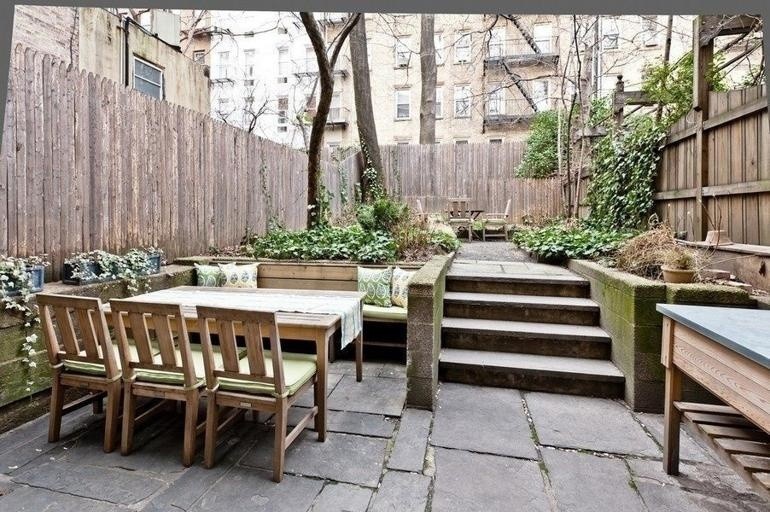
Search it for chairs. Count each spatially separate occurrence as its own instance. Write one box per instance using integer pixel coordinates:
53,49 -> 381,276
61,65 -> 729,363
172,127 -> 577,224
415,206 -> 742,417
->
195,305 -> 320,483
416,200 -> 440,224
482,199 -> 512,241
36,293 -> 163,454
446,198 -> 473,243
108,298 -> 244,467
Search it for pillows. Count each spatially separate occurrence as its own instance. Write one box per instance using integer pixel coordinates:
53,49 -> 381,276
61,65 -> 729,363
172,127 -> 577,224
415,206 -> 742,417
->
217,263 -> 259,291
392,266 -> 418,308
356,266 -> 393,307
192,263 -> 234,287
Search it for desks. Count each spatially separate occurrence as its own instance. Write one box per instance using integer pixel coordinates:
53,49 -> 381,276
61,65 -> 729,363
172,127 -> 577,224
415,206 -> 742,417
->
101,285 -> 368,443
445,210 -> 483,241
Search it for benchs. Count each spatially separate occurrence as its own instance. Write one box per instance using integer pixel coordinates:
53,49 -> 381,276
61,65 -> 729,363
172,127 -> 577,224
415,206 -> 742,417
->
207,262 -> 422,362
